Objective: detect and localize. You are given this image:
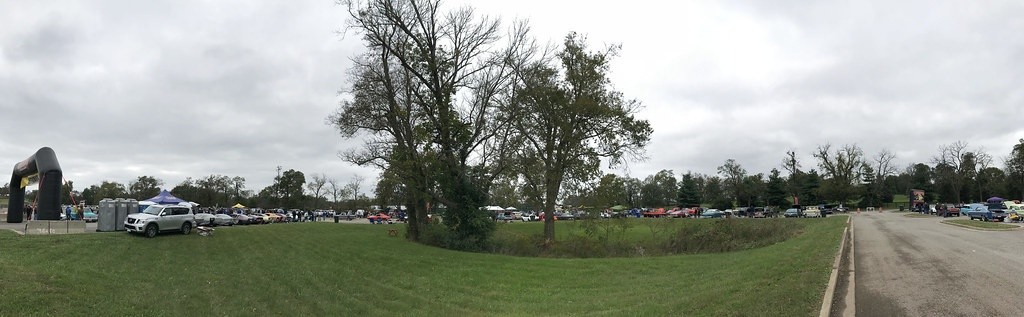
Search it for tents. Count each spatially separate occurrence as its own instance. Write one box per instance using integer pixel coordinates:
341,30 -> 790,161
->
144,190 -> 185,205
987,197 -> 1004,204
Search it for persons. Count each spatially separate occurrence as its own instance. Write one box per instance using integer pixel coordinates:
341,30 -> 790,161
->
66,207 -> 71,220
23,201 -> 1024,222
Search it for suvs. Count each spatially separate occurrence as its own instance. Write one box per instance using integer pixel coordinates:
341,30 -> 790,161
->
123,204 -> 195,238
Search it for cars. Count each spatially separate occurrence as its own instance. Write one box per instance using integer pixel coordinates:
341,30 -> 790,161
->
368,214 -> 390,221
316,210 -> 336,217
197,208 -> 298,227
644,206 -> 767,219
910,188 -> 1024,222
784,202 -> 850,219
71,208 -> 98,222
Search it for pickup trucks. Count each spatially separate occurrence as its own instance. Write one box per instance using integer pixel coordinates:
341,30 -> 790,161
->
334,211 -> 356,221
